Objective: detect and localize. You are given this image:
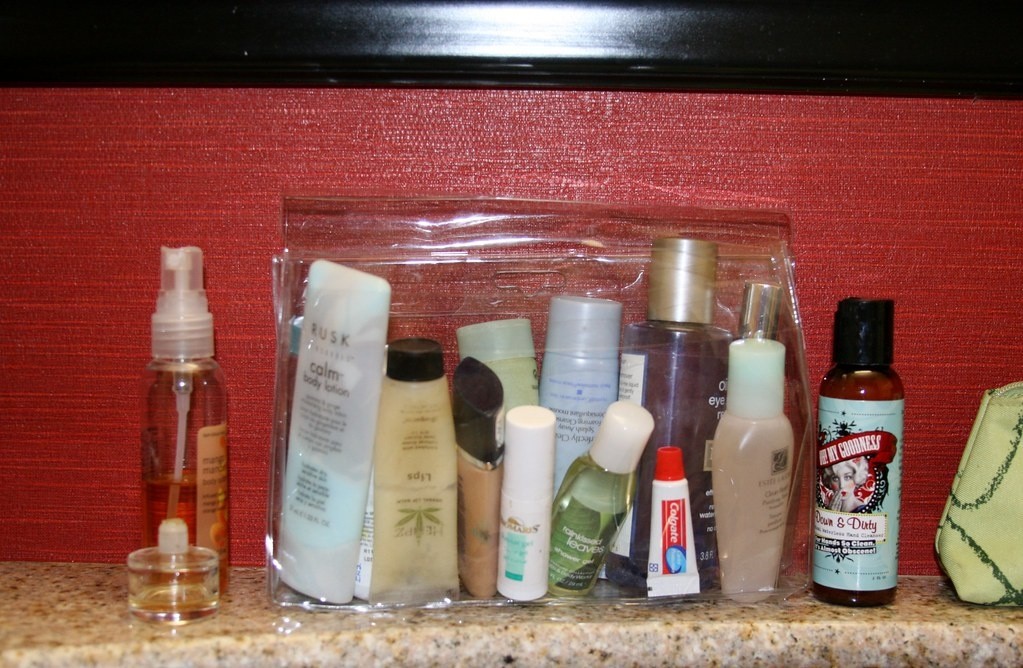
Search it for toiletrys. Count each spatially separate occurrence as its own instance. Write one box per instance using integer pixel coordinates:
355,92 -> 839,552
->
813,299 -> 907,602
124,242 -> 231,619
276,231 -> 793,609
